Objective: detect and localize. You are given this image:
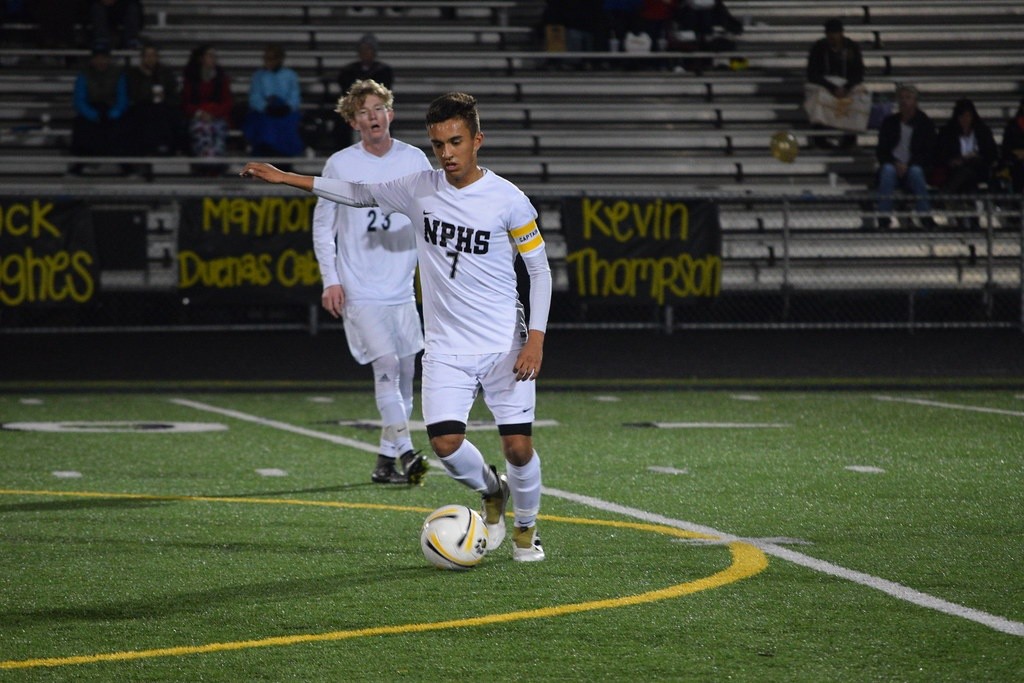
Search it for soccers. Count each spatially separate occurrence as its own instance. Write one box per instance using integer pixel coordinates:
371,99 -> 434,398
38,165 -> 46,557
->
420,504 -> 489,570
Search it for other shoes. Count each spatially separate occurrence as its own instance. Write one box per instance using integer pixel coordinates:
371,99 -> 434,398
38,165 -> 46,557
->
114,162 -> 138,184
911,211 -> 923,228
934,211 -> 949,225
889,215 -> 900,229
980,213 -> 1001,228
57,162 -> 92,182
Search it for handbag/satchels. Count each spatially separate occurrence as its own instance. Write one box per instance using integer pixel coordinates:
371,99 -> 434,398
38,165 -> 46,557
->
610,30 -> 620,51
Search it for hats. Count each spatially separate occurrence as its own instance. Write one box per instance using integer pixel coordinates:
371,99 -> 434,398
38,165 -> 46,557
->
357,31 -> 378,49
94,38 -> 111,54
823,18 -> 845,31
953,98 -> 976,111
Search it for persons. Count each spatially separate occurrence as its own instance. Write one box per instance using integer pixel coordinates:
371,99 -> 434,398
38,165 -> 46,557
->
244,46 -> 304,158
0,0 -> 144,56
312,78 -> 435,484
338,32 -> 392,148
238,93 -> 552,562
132,45 -> 182,151
873,85 -> 1024,230
182,45 -> 232,174
69,39 -> 132,176
531,0 -> 740,76
806,20 -> 865,153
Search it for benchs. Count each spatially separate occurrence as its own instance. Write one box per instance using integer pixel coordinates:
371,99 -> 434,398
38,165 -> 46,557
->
0,0 -> 1024,299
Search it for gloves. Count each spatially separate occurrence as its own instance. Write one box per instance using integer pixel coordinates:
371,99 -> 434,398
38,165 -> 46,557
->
269,103 -> 290,116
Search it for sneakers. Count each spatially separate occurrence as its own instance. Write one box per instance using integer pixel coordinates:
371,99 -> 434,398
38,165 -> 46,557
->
400,449 -> 428,487
510,525 -> 544,562
372,454 -> 409,483
480,464 -> 510,551
